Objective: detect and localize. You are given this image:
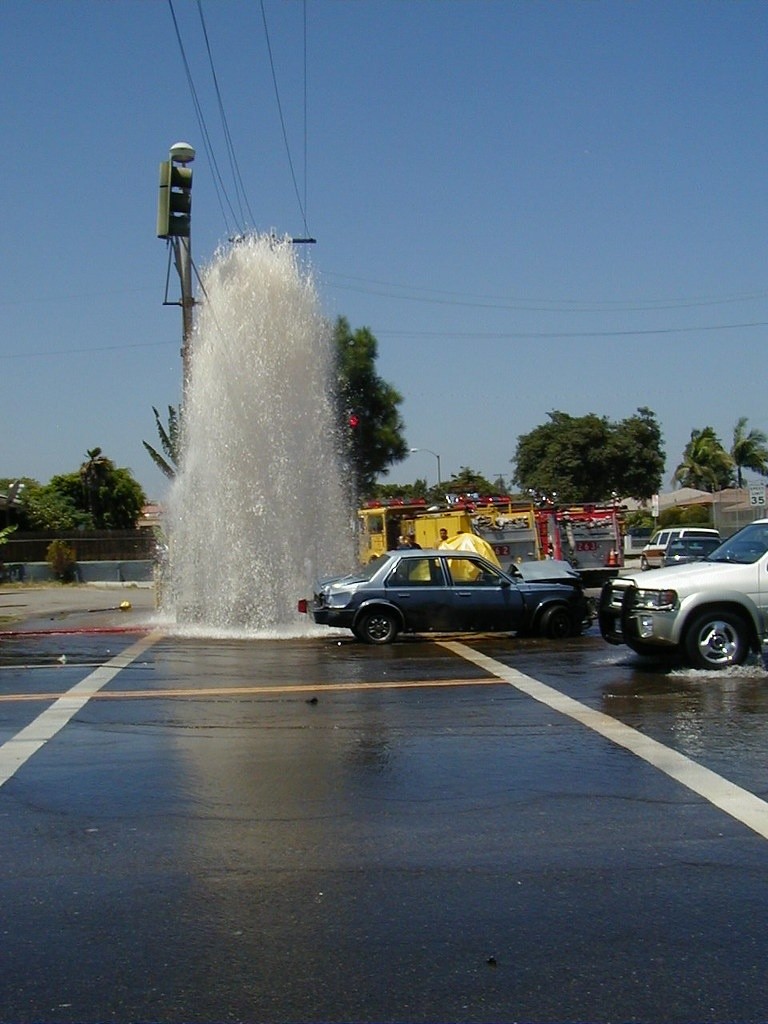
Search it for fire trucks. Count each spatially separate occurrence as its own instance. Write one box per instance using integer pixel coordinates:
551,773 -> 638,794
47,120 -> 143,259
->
357,489 -> 628,589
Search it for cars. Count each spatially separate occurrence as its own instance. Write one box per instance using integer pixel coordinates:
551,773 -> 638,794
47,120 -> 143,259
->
298,549 -> 598,644
658,537 -> 723,568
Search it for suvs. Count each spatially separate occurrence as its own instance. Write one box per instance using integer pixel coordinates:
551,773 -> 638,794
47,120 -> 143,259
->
598,518 -> 768,671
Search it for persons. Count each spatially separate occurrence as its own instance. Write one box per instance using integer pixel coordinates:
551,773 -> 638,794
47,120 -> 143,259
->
155,539 -> 169,580
397,530 -> 422,572
434,528 -> 449,549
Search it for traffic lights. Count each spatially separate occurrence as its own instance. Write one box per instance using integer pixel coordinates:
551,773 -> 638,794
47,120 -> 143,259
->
157,159 -> 193,239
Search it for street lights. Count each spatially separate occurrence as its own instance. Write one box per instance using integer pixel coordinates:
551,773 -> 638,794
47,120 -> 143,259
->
410,448 -> 440,488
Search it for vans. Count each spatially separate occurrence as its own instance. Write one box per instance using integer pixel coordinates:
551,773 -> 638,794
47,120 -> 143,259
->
639,527 -> 722,572
